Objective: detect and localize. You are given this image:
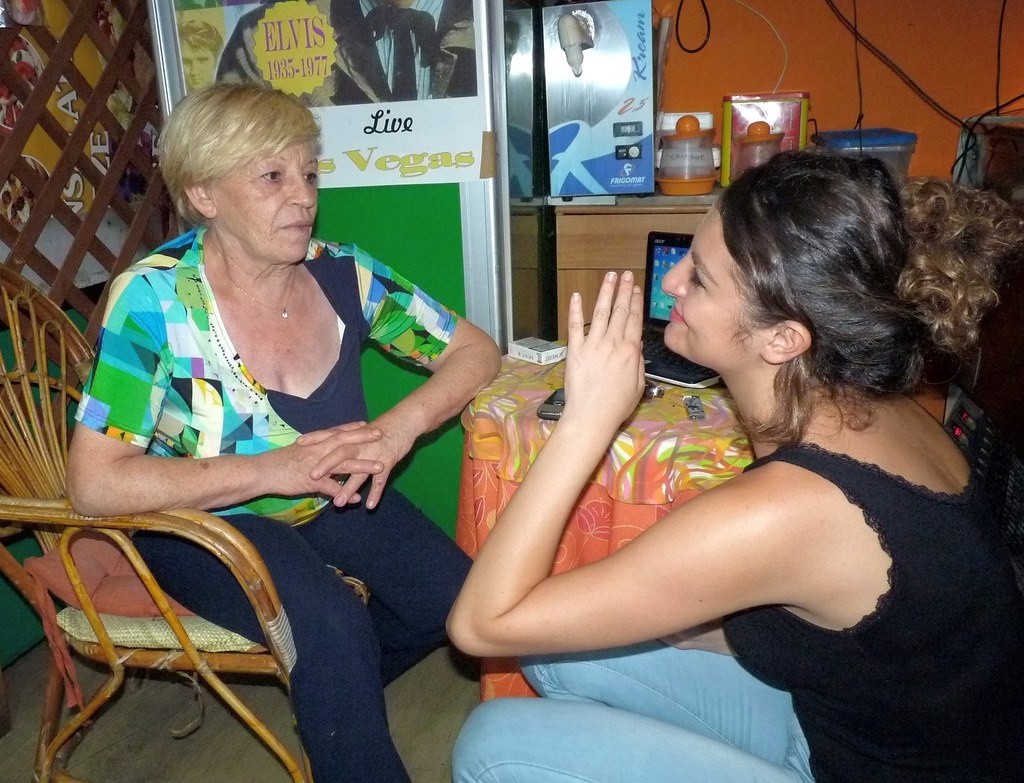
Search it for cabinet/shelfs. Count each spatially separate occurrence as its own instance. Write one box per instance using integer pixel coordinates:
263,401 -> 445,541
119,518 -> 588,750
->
554,186 -> 726,340
503,205 -> 553,341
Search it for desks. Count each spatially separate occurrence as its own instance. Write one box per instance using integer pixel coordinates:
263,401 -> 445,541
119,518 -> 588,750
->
457,340 -> 756,701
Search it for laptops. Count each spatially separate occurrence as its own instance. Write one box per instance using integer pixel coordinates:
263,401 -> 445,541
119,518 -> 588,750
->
643,231 -> 721,389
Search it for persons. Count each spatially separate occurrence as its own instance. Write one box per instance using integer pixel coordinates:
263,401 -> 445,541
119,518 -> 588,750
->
67,83 -> 501,783
445,148 -> 1024,783
178,0 -> 478,109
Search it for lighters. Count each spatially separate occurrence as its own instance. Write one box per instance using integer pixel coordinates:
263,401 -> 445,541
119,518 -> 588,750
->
682,396 -> 705,420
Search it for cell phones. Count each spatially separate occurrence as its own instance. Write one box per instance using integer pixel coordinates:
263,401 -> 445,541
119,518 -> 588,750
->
536,388 -> 565,420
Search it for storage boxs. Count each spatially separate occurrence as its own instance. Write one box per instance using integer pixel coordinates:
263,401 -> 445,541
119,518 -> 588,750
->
810,127 -> 918,179
508,336 -> 567,366
720,92 -> 810,188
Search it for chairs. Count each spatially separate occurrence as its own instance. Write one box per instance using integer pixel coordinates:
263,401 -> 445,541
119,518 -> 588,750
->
0,264 -> 371,783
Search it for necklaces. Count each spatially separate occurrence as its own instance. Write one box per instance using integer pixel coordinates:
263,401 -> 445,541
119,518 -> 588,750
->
225,267 -> 297,318
